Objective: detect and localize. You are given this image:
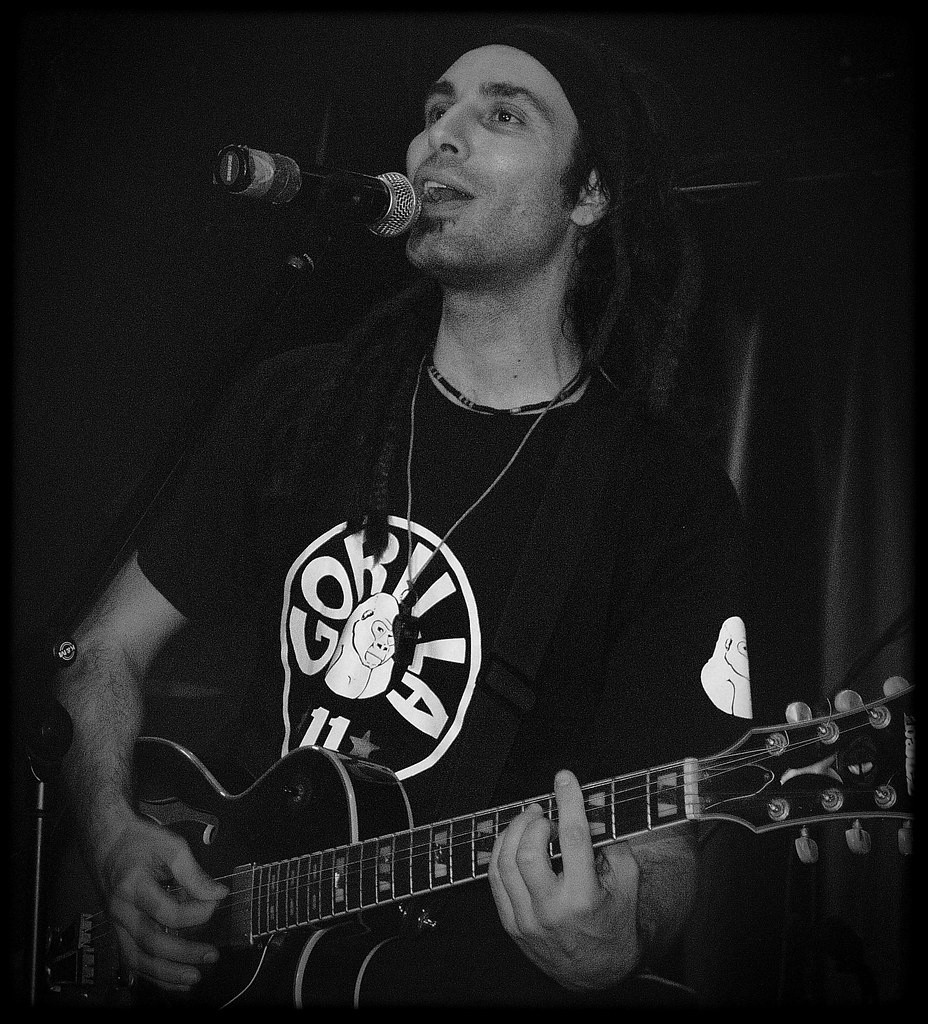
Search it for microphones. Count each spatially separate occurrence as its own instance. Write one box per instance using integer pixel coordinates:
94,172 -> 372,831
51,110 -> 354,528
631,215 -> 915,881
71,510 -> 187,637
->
213,144 -> 423,236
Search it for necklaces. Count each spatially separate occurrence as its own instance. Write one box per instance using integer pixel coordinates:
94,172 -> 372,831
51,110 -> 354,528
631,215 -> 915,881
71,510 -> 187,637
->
428,340 -> 588,410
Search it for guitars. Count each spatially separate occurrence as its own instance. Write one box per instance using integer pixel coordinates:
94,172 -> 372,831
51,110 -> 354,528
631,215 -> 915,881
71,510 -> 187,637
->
0,674 -> 928,1024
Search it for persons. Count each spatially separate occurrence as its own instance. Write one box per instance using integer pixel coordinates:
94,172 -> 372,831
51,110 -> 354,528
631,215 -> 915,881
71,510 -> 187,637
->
59,18 -> 761,1024
